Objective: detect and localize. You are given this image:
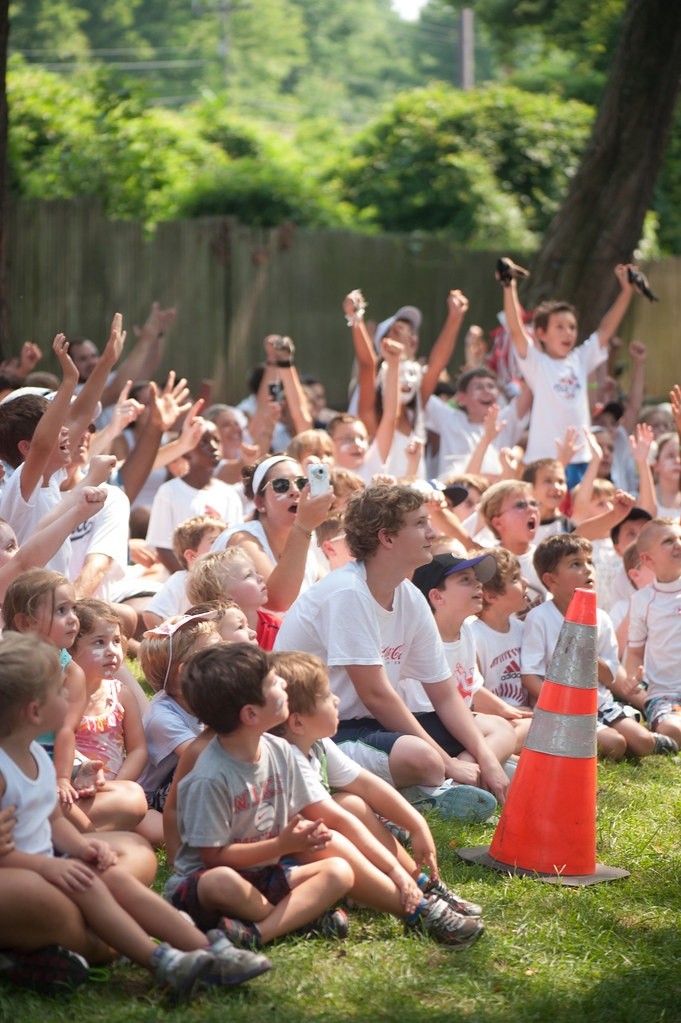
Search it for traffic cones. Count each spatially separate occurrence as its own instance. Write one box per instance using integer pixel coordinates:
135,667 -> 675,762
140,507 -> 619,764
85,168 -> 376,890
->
455,585 -> 630,888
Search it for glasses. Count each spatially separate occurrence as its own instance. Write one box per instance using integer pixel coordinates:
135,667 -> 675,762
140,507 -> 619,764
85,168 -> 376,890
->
499,500 -> 541,517
88,424 -> 95,433
259,476 -> 309,496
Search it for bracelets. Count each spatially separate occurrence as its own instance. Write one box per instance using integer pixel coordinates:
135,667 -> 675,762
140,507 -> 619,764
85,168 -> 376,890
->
275,359 -> 295,368
264,360 -> 277,366
344,309 -> 365,326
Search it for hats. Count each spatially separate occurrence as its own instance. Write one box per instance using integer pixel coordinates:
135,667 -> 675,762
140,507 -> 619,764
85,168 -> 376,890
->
374,305 -> 422,356
44,391 -> 102,422
411,552 -> 498,604
590,400 -> 624,424
427,478 -> 468,508
1,386 -> 52,400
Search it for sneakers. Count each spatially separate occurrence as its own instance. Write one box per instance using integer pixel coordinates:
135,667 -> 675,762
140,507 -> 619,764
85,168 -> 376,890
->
424,879 -> 483,916
217,916 -> 264,953
198,928 -> 272,985
150,941 -> 215,1001
301,906 -> 349,940
408,892 -> 485,951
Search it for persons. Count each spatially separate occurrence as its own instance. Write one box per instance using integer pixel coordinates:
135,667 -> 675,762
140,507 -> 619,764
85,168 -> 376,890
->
0,264 -> 681,1000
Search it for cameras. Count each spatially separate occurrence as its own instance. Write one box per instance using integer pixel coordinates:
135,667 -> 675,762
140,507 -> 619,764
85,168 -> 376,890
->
308,463 -> 330,497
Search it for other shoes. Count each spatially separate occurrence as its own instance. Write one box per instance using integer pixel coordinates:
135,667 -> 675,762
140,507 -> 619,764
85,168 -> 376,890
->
0,944 -> 88,1000
400,777 -> 496,826
651,731 -> 678,756
503,761 -> 518,782
